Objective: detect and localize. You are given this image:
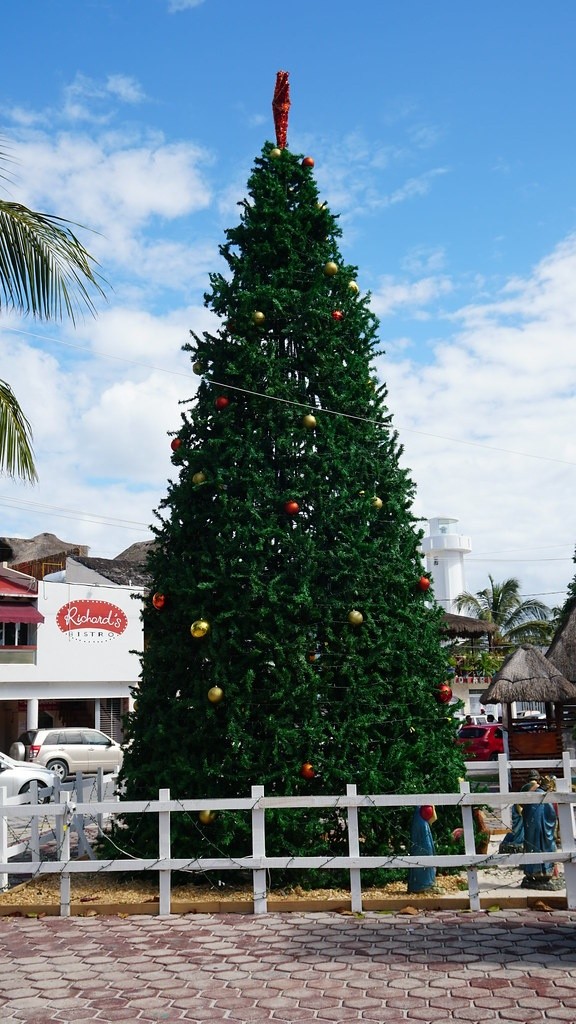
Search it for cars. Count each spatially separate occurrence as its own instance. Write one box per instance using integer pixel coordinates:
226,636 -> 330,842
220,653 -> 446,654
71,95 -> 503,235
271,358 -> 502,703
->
521,714 -> 545,719
453,714 -> 496,732
0,752 -> 59,805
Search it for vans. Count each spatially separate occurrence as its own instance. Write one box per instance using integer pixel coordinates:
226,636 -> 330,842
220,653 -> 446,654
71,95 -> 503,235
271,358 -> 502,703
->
517,710 -> 540,719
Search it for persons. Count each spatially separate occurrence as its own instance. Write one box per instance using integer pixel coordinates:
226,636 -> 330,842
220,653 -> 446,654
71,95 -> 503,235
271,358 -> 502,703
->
461,714 -> 502,729
501,770 -> 558,879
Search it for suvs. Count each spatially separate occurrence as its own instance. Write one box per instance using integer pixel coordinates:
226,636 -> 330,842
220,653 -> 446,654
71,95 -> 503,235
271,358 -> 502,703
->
9,727 -> 125,782
456,723 -> 530,759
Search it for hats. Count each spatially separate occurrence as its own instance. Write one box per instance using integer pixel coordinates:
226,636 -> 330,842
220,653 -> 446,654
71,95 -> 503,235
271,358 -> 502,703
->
481,708 -> 485,712
